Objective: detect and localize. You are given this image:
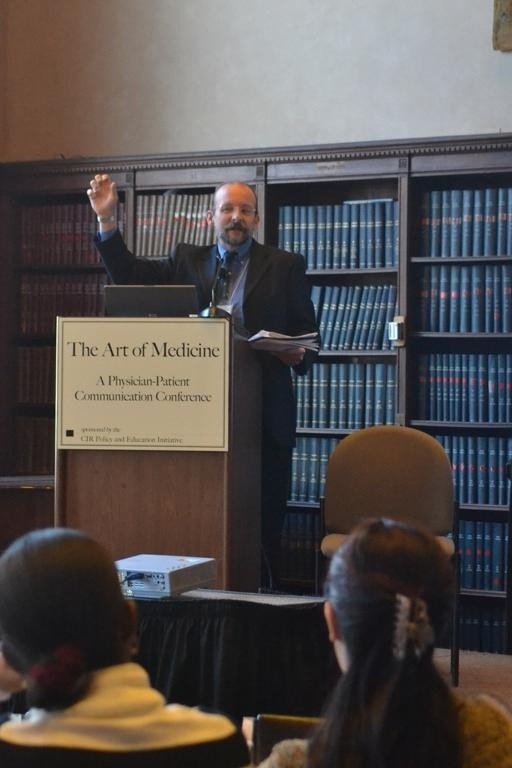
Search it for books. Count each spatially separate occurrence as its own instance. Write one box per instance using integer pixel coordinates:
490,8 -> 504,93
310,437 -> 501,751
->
277,200 -> 399,554
1,202 -> 125,528
136,192 -> 217,258
408,188 -> 512,657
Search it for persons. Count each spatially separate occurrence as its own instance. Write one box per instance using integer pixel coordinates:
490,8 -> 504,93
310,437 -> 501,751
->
251,516 -> 512,767
1,530 -> 251,766
86,175 -> 322,591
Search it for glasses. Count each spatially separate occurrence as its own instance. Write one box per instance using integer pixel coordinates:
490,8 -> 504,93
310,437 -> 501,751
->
218,203 -> 257,216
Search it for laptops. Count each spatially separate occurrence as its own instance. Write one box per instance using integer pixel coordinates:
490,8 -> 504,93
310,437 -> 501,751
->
102,284 -> 201,317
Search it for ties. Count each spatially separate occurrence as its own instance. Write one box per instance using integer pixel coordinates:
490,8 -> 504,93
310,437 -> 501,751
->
213,252 -> 235,304
